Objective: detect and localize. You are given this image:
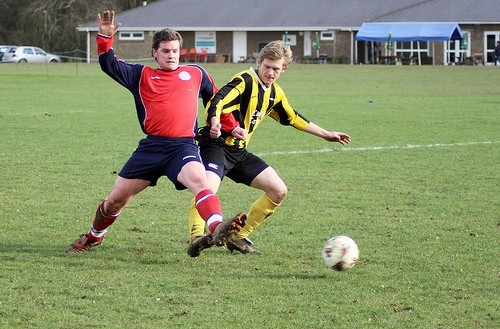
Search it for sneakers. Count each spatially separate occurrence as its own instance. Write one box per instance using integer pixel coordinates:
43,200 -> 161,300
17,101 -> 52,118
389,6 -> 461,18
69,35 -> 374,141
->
187,233 -> 214,258
226,237 -> 261,256
64,233 -> 105,255
214,212 -> 248,247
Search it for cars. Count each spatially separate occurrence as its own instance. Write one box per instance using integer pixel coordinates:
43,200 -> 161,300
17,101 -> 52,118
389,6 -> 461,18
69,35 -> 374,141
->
0,46 -> 61,63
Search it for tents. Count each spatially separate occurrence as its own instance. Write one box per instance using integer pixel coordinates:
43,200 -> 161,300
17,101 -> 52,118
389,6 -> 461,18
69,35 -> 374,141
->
354,21 -> 463,65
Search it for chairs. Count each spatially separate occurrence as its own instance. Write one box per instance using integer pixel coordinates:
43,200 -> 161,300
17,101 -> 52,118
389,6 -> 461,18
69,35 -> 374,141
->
292,54 -> 349,64
375,55 -> 419,66
180,49 -> 208,63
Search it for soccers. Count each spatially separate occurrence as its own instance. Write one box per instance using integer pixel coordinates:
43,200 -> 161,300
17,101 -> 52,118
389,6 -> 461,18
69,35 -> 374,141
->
321,235 -> 360,272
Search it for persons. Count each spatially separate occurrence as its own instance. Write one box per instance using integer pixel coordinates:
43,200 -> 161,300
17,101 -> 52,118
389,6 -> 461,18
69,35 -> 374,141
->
187,40 -> 352,258
66,10 -> 249,254
494,41 -> 500,66
